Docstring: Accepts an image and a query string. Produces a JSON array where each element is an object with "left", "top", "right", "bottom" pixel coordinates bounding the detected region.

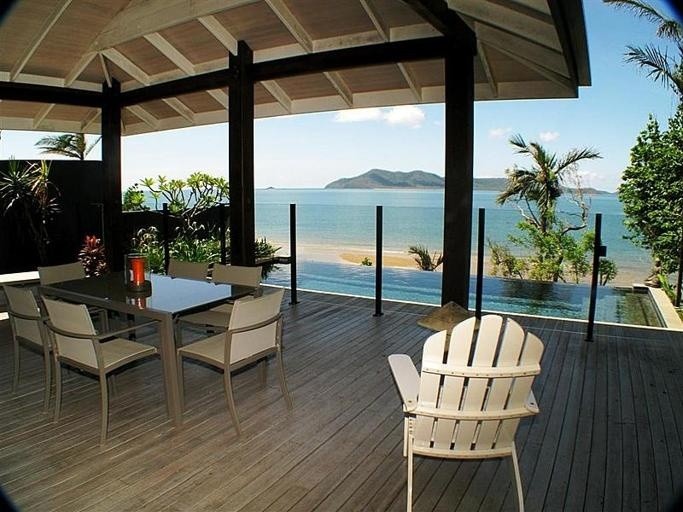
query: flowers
[{"left": 79, "top": 235, "right": 107, "bottom": 280}]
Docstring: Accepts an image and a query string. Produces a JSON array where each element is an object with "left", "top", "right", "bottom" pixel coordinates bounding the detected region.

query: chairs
[
  {"left": 176, "top": 286, "right": 291, "bottom": 438},
  {"left": 37, "top": 260, "right": 105, "bottom": 338},
  {"left": 386, "top": 314, "right": 544, "bottom": 512},
  {"left": 2, "top": 283, "right": 112, "bottom": 414},
  {"left": 39, "top": 294, "right": 168, "bottom": 445},
  {"left": 178, "top": 264, "right": 267, "bottom": 389},
  {"left": 166, "top": 257, "right": 212, "bottom": 348}
]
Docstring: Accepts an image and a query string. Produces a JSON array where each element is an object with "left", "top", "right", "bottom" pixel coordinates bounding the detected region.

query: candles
[{"left": 133, "top": 261, "right": 145, "bottom": 284}]
